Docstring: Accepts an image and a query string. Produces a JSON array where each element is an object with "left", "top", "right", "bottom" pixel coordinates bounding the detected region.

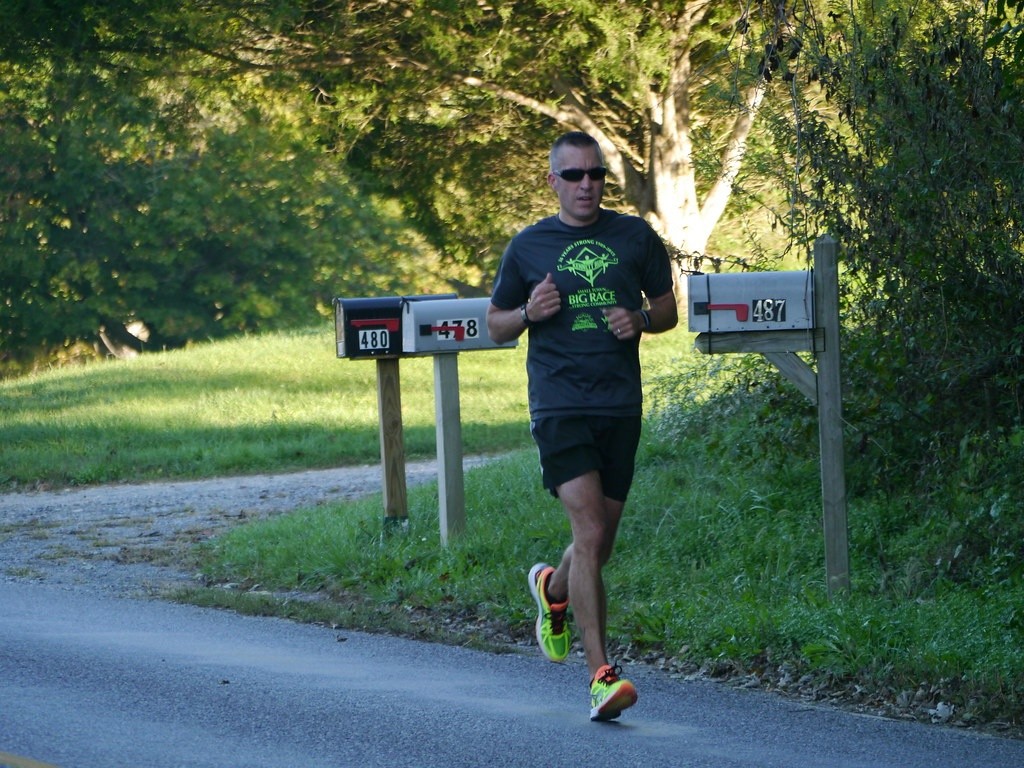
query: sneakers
[
  {"left": 528, "top": 563, "right": 571, "bottom": 662},
  {"left": 590, "top": 660, "right": 637, "bottom": 722}
]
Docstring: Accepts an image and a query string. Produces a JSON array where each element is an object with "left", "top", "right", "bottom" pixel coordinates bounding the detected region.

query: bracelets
[{"left": 638, "top": 309, "right": 651, "bottom": 331}]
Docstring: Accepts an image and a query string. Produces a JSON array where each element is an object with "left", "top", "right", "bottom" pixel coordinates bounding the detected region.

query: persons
[{"left": 485, "top": 131, "right": 679, "bottom": 722}]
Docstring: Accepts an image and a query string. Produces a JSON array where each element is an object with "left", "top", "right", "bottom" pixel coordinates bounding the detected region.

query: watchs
[{"left": 520, "top": 303, "right": 539, "bottom": 326}]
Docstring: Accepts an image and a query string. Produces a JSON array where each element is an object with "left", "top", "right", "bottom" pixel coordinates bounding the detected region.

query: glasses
[{"left": 552, "top": 167, "right": 607, "bottom": 181}]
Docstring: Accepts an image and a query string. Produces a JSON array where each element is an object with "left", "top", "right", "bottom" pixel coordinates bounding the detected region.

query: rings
[{"left": 617, "top": 328, "right": 621, "bottom": 334}]
